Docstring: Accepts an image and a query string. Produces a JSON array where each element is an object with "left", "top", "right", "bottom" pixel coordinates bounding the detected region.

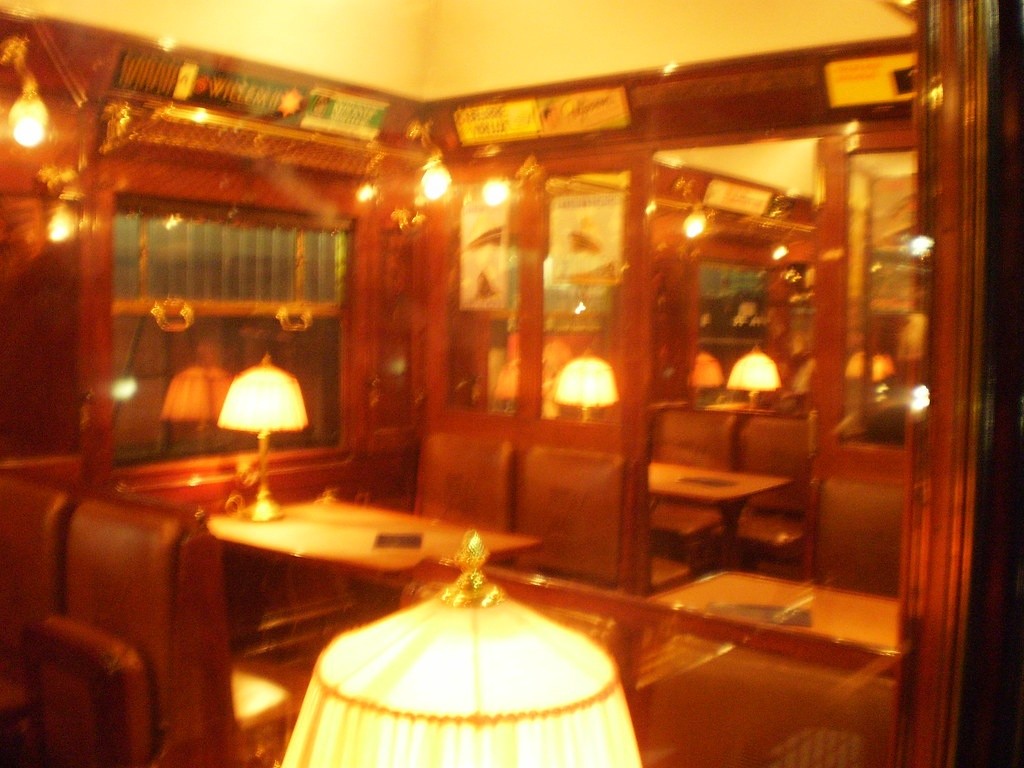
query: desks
[
  {"left": 206, "top": 495, "right": 541, "bottom": 667},
  {"left": 647, "top": 459, "right": 795, "bottom": 571},
  {"left": 615, "top": 572, "right": 902, "bottom": 768}
]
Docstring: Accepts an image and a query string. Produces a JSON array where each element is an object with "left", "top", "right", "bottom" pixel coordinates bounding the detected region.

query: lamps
[
  {"left": 162, "top": 366, "right": 230, "bottom": 454},
  {"left": 729, "top": 347, "right": 779, "bottom": 411},
  {"left": 693, "top": 352, "right": 722, "bottom": 391},
  {"left": 10, "top": 95, "right": 47, "bottom": 149},
  {"left": 553, "top": 356, "right": 617, "bottom": 425},
  {"left": 277, "top": 530, "right": 645, "bottom": 768},
  {"left": 422, "top": 158, "right": 451, "bottom": 201},
  {"left": 218, "top": 355, "right": 308, "bottom": 523}
]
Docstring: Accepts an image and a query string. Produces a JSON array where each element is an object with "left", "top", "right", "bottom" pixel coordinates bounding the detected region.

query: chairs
[
  {"left": 739, "top": 415, "right": 817, "bottom": 580},
  {"left": 7, "top": 617, "right": 150, "bottom": 767},
  {"left": 414, "top": 431, "right": 513, "bottom": 535},
  {"left": 0, "top": 473, "right": 67, "bottom": 715},
  {"left": 648, "top": 411, "right": 735, "bottom": 577},
  {"left": 519, "top": 445, "right": 621, "bottom": 587},
  {"left": 60, "top": 492, "right": 290, "bottom": 767}
]
